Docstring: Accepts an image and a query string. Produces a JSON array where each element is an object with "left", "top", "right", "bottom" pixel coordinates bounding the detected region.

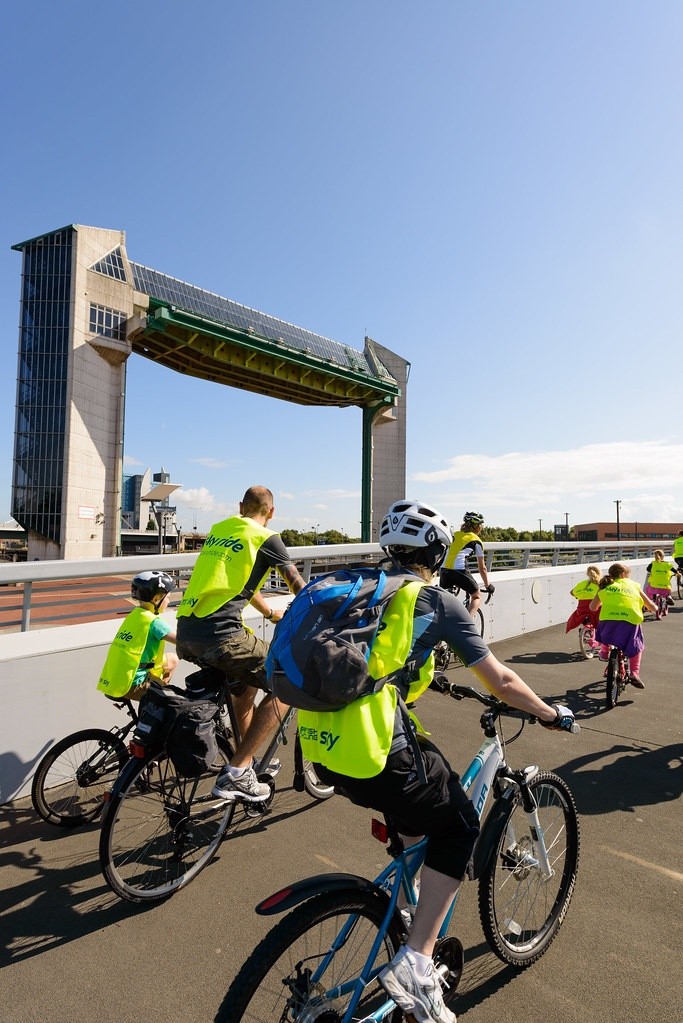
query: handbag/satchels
[{"left": 135, "top": 680, "right": 220, "bottom": 777}]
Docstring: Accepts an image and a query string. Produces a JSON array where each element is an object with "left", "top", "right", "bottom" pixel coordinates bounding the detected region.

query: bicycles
[
  {"left": 678, "top": 565, "right": 683, "bottom": 600},
  {"left": 605, "top": 645, "right": 637, "bottom": 708},
  {"left": 656, "top": 573, "right": 678, "bottom": 620},
  {"left": 580, "top": 620, "right": 600, "bottom": 660},
  {"left": 215, "top": 681, "right": 581, "bottom": 1023},
  {"left": 31, "top": 686, "right": 336, "bottom": 906},
  {"left": 435, "top": 588, "right": 492, "bottom": 672}
]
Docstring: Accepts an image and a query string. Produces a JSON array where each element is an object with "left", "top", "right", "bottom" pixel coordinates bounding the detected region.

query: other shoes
[
  {"left": 630, "top": 676, "right": 645, "bottom": 689},
  {"left": 666, "top": 597, "right": 674, "bottom": 606}
]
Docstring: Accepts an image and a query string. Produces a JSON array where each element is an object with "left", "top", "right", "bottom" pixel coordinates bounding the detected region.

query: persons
[
  {"left": 439, "top": 511, "right": 495, "bottom": 619},
  {"left": 96, "top": 571, "right": 179, "bottom": 704},
  {"left": 570, "top": 567, "right": 604, "bottom": 648},
  {"left": 590, "top": 564, "right": 658, "bottom": 689},
  {"left": 297, "top": 500, "right": 574, "bottom": 1023},
  {"left": 174, "top": 486, "right": 306, "bottom": 802},
  {"left": 646, "top": 550, "right": 682, "bottom": 602},
  {"left": 673, "top": 530, "right": 683, "bottom": 572}
]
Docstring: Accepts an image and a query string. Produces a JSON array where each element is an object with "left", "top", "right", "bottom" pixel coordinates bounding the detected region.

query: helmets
[
  {"left": 464, "top": 511, "right": 484, "bottom": 523},
  {"left": 131, "top": 571, "right": 177, "bottom": 601},
  {"left": 379, "top": 499, "right": 454, "bottom": 547}
]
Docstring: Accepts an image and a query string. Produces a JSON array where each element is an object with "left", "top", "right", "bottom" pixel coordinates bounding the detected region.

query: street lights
[
  {"left": 312, "top": 523, "right": 320, "bottom": 545},
  {"left": 302, "top": 530, "right": 305, "bottom": 546}
]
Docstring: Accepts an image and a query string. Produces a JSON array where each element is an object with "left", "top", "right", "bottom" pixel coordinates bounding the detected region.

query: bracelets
[{"left": 264, "top": 609, "right": 274, "bottom": 619}]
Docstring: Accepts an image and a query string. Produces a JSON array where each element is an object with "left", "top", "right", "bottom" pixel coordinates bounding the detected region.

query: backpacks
[{"left": 266, "top": 569, "right": 434, "bottom": 711}]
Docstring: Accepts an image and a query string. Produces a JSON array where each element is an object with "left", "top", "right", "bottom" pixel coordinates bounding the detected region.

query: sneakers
[
  {"left": 211, "top": 765, "right": 272, "bottom": 802},
  {"left": 378, "top": 945, "right": 457, "bottom": 1023},
  {"left": 399, "top": 880, "right": 419, "bottom": 935},
  {"left": 253, "top": 758, "right": 281, "bottom": 777}
]
